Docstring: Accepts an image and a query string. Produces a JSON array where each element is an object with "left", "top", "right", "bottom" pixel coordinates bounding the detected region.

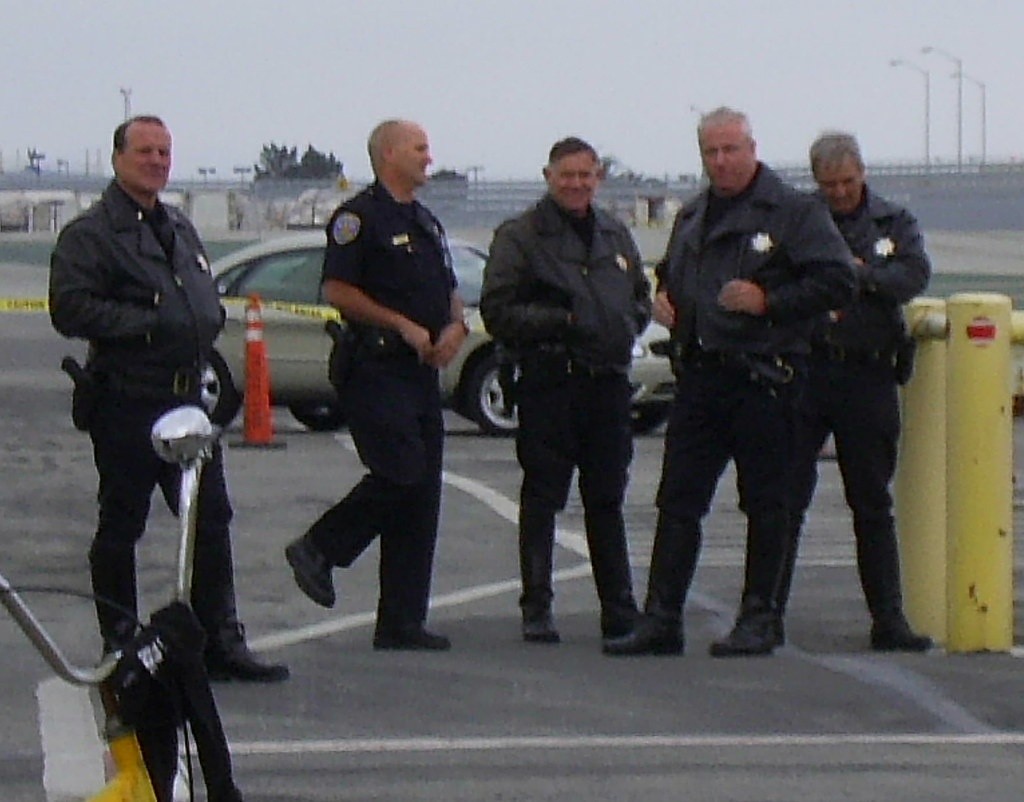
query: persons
[
  {"left": 48, "top": 114, "right": 290, "bottom": 683},
  {"left": 284, "top": 117, "right": 464, "bottom": 652},
  {"left": 770, "top": 131, "right": 935, "bottom": 657},
  {"left": 604, "top": 107, "right": 859, "bottom": 659},
  {"left": 482, "top": 136, "right": 651, "bottom": 656}
]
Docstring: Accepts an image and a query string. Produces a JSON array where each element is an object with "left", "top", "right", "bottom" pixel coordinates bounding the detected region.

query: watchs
[{"left": 452, "top": 318, "right": 471, "bottom": 334}]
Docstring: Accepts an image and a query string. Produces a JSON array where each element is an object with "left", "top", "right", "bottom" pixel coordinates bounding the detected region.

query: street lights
[
  {"left": 199, "top": 168, "right": 216, "bottom": 183},
  {"left": 892, "top": 60, "right": 930, "bottom": 174},
  {"left": 950, "top": 73, "right": 986, "bottom": 173},
  {"left": 234, "top": 168, "right": 251, "bottom": 189},
  {"left": 923, "top": 47, "right": 962, "bottom": 173}
]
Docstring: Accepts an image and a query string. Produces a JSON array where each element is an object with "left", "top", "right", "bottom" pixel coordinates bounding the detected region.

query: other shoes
[
  {"left": 522, "top": 606, "right": 560, "bottom": 643},
  {"left": 207, "top": 641, "right": 289, "bottom": 682},
  {"left": 604, "top": 626, "right": 684, "bottom": 656},
  {"left": 287, "top": 533, "right": 339, "bottom": 608},
  {"left": 871, "top": 631, "right": 933, "bottom": 653},
  {"left": 373, "top": 624, "right": 451, "bottom": 651},
  {"left": 600, "top": 611, "right": 639, "bottom": 636},
  {"left": 709, "top": 629, "right": 776, "bottom": 658}
]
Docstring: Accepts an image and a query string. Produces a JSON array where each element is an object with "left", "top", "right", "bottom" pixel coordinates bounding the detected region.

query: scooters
[{"left": 1, "top": 404, "right": 213, "bottom": 802}]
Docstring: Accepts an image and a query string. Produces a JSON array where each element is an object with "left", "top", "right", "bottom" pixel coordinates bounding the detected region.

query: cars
[{"left": 200, "top": 231, "right": 677, "bottom": 439}]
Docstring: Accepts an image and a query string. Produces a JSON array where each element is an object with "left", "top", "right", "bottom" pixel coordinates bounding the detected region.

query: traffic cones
[{"left": 226, "top": 294, "right": 286, "bottom": 449}]
